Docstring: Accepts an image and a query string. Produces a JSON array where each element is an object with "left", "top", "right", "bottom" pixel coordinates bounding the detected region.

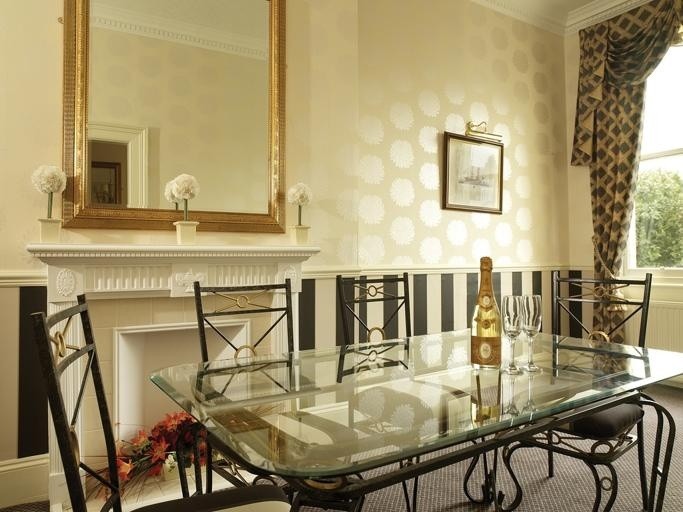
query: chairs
[
  {"left": 28, "top": 293, "right": 296, "bottom": 512},
  {"left": 495, "top": 269, "right": 652, "bottom": 512},
  {"left": 193, "top": 280, "right": 365, "bottom": 512},
  {"left": 339, "top": 273, "right": 494, "bottom": 512}
]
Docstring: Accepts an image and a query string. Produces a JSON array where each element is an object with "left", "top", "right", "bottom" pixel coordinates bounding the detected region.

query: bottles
[{"left": 469, "top": 255, "right": 502, "bottom": 371}]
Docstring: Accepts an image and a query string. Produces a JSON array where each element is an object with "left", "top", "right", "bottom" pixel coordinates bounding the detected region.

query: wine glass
[
  {"left": 521, "top": 294, "right": 543, "bottom": 373},
  {"left": 500, "top": 295, "right": 523, "bottom": 376}
]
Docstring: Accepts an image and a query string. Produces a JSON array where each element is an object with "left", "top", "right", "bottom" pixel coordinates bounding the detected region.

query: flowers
[
  {"left": 164, "top": 179, "right": 183, "bottom": 211},
  {"left": 173, "top": 173, "right": 198, "bottom": 220},
  {"left": 287, "top": 183, "right": 312, "bottom": 226},
  {"left": 30, "top": 164, "right": 67, "bottom": 217}
]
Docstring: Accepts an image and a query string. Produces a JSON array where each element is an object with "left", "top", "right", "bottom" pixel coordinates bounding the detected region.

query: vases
[
  {"left": 172, "top": 220, "right": 199, "bottom": 246},
  {"left": 35, "top": 218, "right": 64, "bottom": 244},
  {"left": 290, "top": 226, "right": 311, "bottom": 244}
]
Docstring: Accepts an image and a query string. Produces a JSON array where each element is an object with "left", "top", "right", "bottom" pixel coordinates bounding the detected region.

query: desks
[{"left": 148, "top": 328, "right": 683, "bottom": 512}]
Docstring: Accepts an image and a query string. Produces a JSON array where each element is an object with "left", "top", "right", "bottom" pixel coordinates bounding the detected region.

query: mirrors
[{"left": 61, "top": 0, "right": 287, "bottom": 234}]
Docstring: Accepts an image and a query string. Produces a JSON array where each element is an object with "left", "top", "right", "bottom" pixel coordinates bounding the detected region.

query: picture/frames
[
  {"left": 440, "top": 131, "right": 504, "bottom": 217},
  {"left": 90, "top": 161, "right": 121, "bottom": 204}
]
